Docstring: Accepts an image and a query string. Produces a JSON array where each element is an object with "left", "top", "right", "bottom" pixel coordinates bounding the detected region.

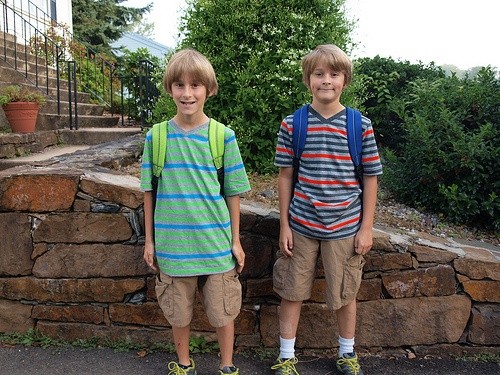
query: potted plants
[{"left": 0, "top": 84, "right": 44, "bottom": 133}]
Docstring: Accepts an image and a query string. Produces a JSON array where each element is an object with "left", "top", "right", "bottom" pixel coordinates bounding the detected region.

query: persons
[
  {"left": 270, "top": 45, "right": 383, "bottom": 375},
  {"left": 140, "top": 49, "right": 251, "bottom": 375}
]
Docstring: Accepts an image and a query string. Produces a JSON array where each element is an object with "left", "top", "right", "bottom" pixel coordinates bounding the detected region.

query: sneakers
[
  {"left": 217, "top": 360, "right": 240, "bottom": 375},
  {"left": 168, "top": 357, "right": 197, "bottom": 374},
  {"left": 336, "top": 352, "right": 364, "bottom": 375},
  {"left": 271, "top": 356, "right": 299, "bottom": 374}
]
